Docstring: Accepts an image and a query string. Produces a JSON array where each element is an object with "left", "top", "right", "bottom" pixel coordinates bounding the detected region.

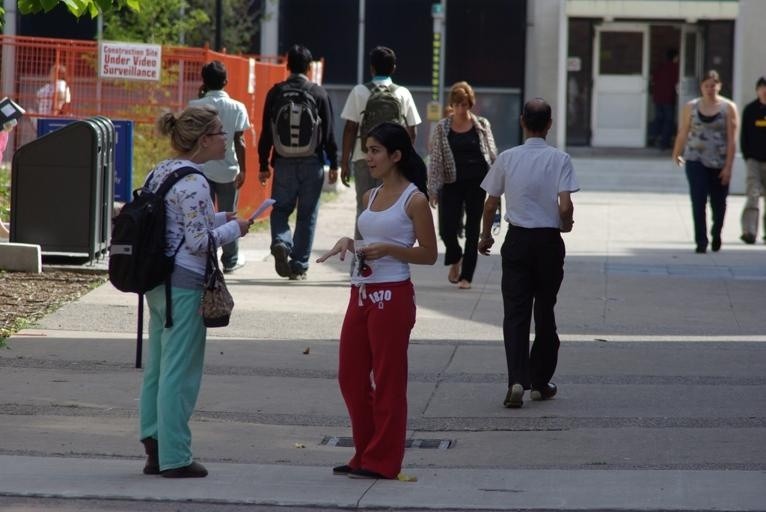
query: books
[{"left": 0, "top": 96, "right": 25, "bottom": 131}]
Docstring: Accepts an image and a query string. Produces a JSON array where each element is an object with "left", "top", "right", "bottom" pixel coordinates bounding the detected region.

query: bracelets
[{"left": 479, "top": 233, "right": 492, "bottom": 242}]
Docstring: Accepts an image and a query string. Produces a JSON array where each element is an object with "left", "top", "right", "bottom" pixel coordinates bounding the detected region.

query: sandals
[{"left": 448, "top": 255, "right": 464, "bottom": 284}]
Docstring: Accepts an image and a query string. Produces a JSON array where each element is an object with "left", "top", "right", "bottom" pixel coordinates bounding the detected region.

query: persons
[
  {"left": 314, "top": 122, "right": 436, "bottom": 481},
  {"left": 429, "top": 81, "right": 496, "bottom": 289},
  {"left": 257, "top": 45, "right": 338, "bottom": 281},
  {"left": 673, "top": 69, "right": 737, "bottom": 254},
  {"left": 27, "top": 63, "right": 71, "bottom": 131},
  {"left": 0, "top": 117, "right": 17, "bottom": 167},
  {"left": 136, "top": 105, "right": 251, "bottom": 479},
  {"left": 738, "top": 75, "right": 766, "bottom": 245},
  {"left": 339, "top": 46, "right": 421, "bottom": 242},
  {"left": 184, "top": 60, "right": 250, "bottom": 274},
  {"left": 478, "top": 98, "right": 580, "bottom": 409}
]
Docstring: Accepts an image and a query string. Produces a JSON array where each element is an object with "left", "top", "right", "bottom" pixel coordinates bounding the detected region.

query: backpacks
[
  {"left": 109, "top": 167, "right": 204, "bottom": 293},
  {"left": 360, "top": 80, "right": 404, "bottom": 151},
  {"left": 268, "top": 80, "right": 323, "bottom": 159}
]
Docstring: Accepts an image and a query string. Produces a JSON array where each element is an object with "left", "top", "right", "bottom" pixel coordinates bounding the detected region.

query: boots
[{"left": 143, "top": 438, "right": 208, "bottom": 477}]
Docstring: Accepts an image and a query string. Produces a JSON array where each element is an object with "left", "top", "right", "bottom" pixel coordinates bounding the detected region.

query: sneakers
[
  {"left": 332, "top": 464, "right": 385, "bottom": 479},
  {"left": 504, "top": 382, "right": 524, "bottom": 408},
  {"left": 223, "top": 256, "right": 247, "bottom": 272},
  {"left": 531, "top": 382, "right": 557, "bottom": 399},
  {"left": 272, "top": 241, "right": 307, "bottom": 280}
]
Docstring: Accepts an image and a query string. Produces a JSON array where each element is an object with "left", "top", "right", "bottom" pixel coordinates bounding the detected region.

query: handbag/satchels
[{"left": 200, "top": 229, "right": 233, "bottom": 327}]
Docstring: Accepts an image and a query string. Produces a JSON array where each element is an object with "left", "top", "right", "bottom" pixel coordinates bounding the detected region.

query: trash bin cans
[{"left": 8, "top": 116, "right": 117, "bottom": 256}]
